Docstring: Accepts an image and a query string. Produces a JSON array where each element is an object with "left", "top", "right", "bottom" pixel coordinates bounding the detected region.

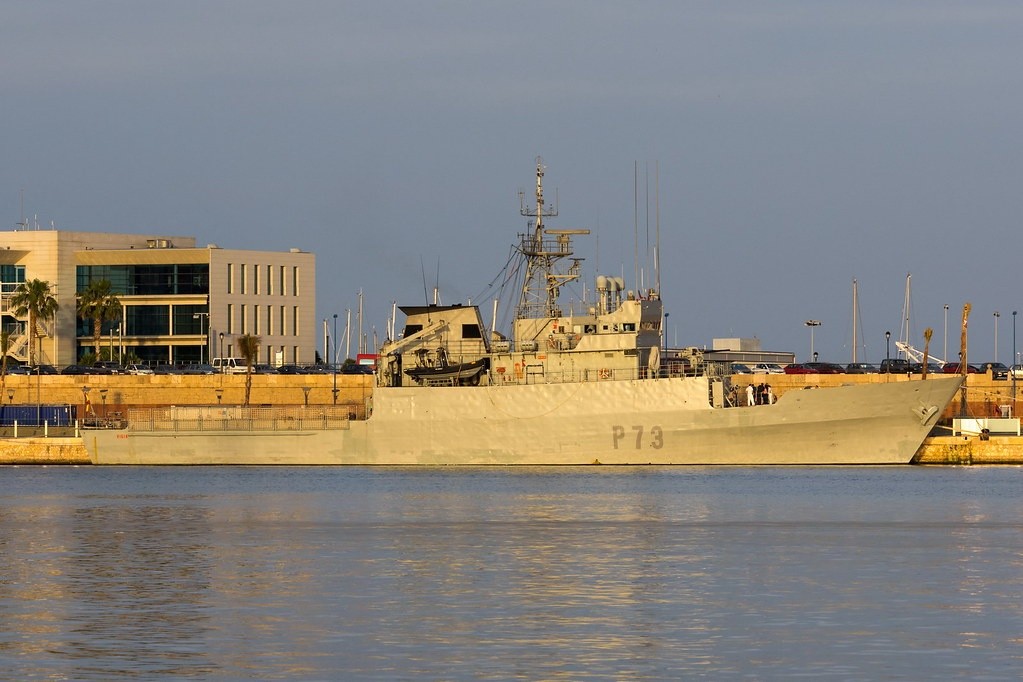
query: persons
[{"left": 745, "top": 383, "right": 772, "bottom": 406}]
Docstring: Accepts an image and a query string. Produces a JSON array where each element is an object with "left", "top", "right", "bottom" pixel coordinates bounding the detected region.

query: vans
[{"left": 212, "top": 358, "right": 256, "bottom": 374}]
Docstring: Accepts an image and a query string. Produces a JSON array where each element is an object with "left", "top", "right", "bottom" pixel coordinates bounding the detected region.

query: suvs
[
  {"left": 879, "top": 358, "right": 914, "bottom": 374},
  {"left": 92, "top": 361, "right": 130, "bottom": 375}
]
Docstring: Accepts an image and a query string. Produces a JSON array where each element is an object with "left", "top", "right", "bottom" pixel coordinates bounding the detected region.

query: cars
[
  {"left": 731, "top": 363, "right": 754, "bottom": 375},
  {"left": 124, "top": 364, "right": 156, "bottom": 375},
  {"left": 61, "top": 364, "right": 100, "bottom": 375},
  {"left": 1010, "top": 364, "right": 1023, "bottom": 379},
  {"left": 979, "top": 362, "right": 1011, "bottom": 380},
  {"left": 154, "top": 365, "right": 184, "bottom": 375},
  {"left": 277, "top": 366, "right": 308, "bottom": 375},
  {"left": 252, "top": 365, "right": 280, "bottom": 374},
  {"left": 4, "top": 366, "right": 38, "bottom": 375},
  {"left": 846, "top": 363, "right": 877, "bottom": 373},
  {"left": 942, "top": 363, "right": 979, "bottom": 375},
  {"left": 303, "top": 364, "right": 344, "bottom": 374},
  {"left": 784, "top": 362, "right": 846, "bottom": 375},
  {"left": 340, "top": 364, "right": 376, "bottom": 374},
  {"left": 751, "top": 363, "right": 786, "bottom": 374},
  {"left": 912, "top": 362, "right": 942, "bottom": 373},
  {"left": 182, "top": 364, "right": 220, "bottom": 375},
  {"left": 29, "top": 365, "right": 60, "bottom": 374}
]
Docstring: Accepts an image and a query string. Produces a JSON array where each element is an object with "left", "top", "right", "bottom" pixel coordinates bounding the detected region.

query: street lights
[
  {"left": 993, "top": 311, "right": 1000, "bottom": 362},
  {"left": 219, "top": 331, "right": 224, "bottom": 374},
  {"left": 194, "top": 313, "right": 211, "bottom": 365},
  {"left": 806, "top": 319, "right": 822, "bottom": 363},
  {"left": 943, "top": 303, "right": 949, "bottom": 362},
  {"left": 664, "top": 313, "right": 669, "bottom": 366},
  {"left": 332, "top": 314, "right": 338, "bottom": 406},
  {"left": 1012, "top": 311, "right": 1017, "bottom": 416}
]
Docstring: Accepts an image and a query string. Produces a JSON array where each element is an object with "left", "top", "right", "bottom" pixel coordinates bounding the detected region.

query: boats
[
  {"left": 402, "top": 355, "right": 491, "bottom": 381},
  {"left": 76, "top": 154, "right": 970, "bottom": 466}
]
